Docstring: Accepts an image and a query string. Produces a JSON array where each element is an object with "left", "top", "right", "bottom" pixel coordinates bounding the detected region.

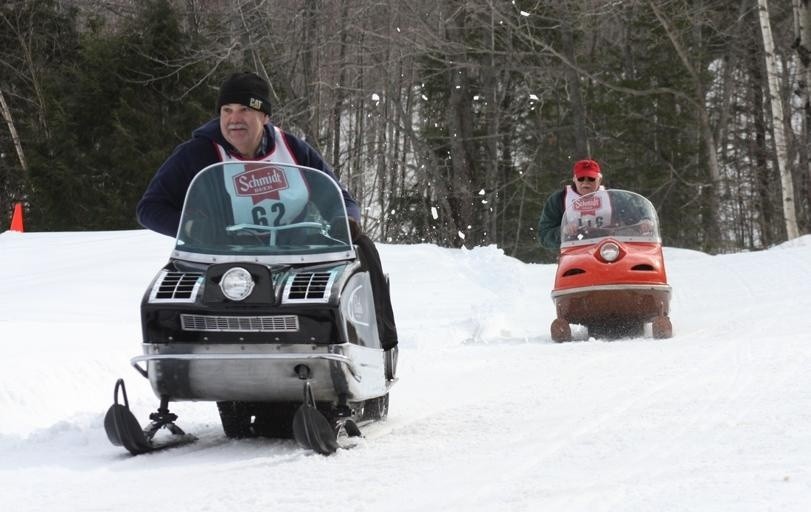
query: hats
[
  {"left": 219, "top": 72, "right": 273, "bottom": 115},
  {"left": 574, "top": 159, "right": 601, "bottom": 178}
]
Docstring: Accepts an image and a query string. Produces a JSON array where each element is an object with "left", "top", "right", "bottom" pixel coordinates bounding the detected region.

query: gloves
[
  {"left": 191, "top": 221, "right": 224, "bottom": 246},
  {"left": 329, "top": 217, "right": 361, "bottom": 246}
]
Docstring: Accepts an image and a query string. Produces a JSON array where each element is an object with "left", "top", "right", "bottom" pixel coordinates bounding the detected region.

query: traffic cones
[{"left": 10, "top": 202, "right": 24, "bottom": 232}]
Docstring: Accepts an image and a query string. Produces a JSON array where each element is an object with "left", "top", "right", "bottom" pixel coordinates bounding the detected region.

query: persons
[
  {"left": 135, "top": 71, "right": 364, "bottom": 246},
  {"left": 535, "top": 160, "right": 655, "bottom": 252}
]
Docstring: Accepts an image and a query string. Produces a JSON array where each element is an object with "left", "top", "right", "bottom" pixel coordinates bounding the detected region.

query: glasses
[{"left": 578, "top": 177, "right": 595, "bottom": 183}]
